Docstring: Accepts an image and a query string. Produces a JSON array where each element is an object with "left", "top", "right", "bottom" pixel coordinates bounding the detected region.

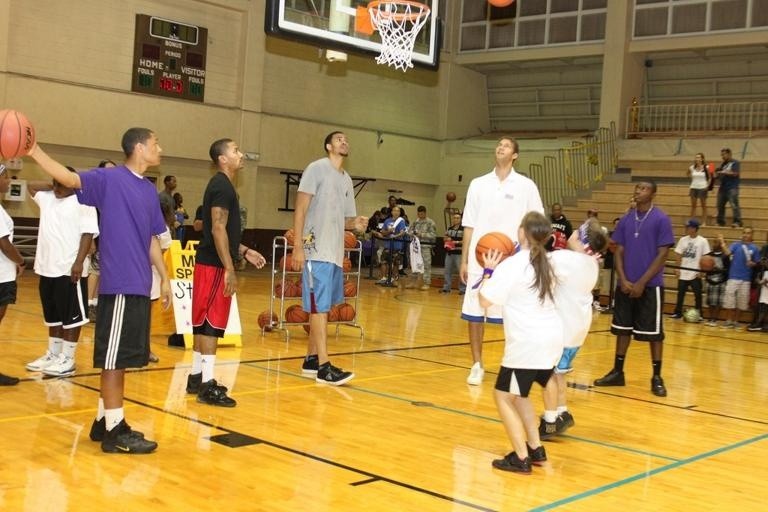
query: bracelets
[
  {"left": 242, "top": 248, "right": 250, "bottom": 260},
  {"left": 20, "top": 258, "right": 26, "bottom": 267},
  {"left": 482, "top": 267, "right": 493, "bottom": 280}
]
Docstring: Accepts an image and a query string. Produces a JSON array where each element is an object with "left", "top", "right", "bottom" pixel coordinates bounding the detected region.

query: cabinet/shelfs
[{"left": 261, "top": 234, "right": 367, "bottom": 341}]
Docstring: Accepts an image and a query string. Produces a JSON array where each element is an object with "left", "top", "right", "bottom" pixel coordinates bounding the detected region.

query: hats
[{"left": 685, "top": 219, "right": 699, "bottom": 226}]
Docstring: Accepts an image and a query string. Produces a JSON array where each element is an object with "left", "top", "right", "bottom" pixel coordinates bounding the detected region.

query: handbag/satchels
[{"left": 704, "top": 163, "right": 714, "bottom": 191}]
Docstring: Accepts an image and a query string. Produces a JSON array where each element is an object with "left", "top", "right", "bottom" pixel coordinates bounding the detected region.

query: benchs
[{"left": 555, "top": 159, "right": 768, "bottom": 324}]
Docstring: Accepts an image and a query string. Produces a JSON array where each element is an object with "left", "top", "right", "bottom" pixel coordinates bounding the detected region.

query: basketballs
[
  {"left": 0, "top": 109, "right": 36, "bottom": 160},
  {"left": 488, "top": 0, "right": 514, "bottom": 8},
  {"left": 258, "top": 228, "right": 357, "bottom": 335},
  {"left": 700, "top": 256, "right": 715, "bottom": 270},
  {"left": 683, "top": 308, "right": 701, "bottom": 323},
  {"left": 475, "top": 233, "right": 514, "bottom": 268},
  {"left": 446, "top": 192, "right": 456, "bottom": 202}
]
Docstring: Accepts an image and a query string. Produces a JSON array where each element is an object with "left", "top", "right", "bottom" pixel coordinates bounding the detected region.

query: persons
[
  {"left": 25, "top": 158, "right": 203, "bottom": 377},
  {"left": 593, "top": 179, "right": 676, "bottom": 397},
  {"left": 687, "top": 152, "right": 714, "bottom": 227},
  {"left": 674, "top": 219, "right": 768, "bottom": 334},
  {"left": 290, "top": 128, "right": 369, "bottom": 384},
  {"left": 537, "top": 216, "right": 609, "bottom": 439},
  {"left": 713, "top": 148, "right": 744, "bottom": 227},
  {"left": 549, "top": 201, "right": 620, "bottom": 311},
  {"left": 24, "top": 125, "right": 176, "bottom": 457},
  {"left": 477, "top": 211, "right": 566, "bottom": 475},
  {"left": 23, "top": 372, "right": 86, "bottom": 512},
  {"left": 663, "top": 327, "right": 768, "bottom": 444},
  {"left": 368, "top": 195, "right": 466, "bottom": 295},
  {"left": 458, "top": 135, "right": 547, "bottom": 385},
  {"left": 0, "top": 163, "right": 25, "bottom": 383},
  {"left": 186, "top": 139, "right": 266, "bottom": 406}
]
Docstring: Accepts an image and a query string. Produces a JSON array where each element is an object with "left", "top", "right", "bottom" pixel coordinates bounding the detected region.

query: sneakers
[
  {"left": 539, "top": 411, "right": 574, "bottom": 440},
  {"left": 186, "top": 372, "right": 228, "bottom": 394},
  {"left": 0, "top": 352, "right": 77, "bottom": 386},
  {"left": 671, "top": 314, "right": 767, "bottom": 332},
  {"left": 198, "top": 379, "right": 236, "bottom": 407},
  {"left": 301, "top": 358, "right": 319, "bottom": 374},
  {"left": 594, "top": 369, "right": 625, "bottom": 386},
  {"left": 88, "top": 304, "right": 98, "bottom": 322},
  {"left": 491, "top": 442, "right": 547, "bottom": 475},
  {"left": 651, "top": 375, "right": 666, "bottom": 396},
  {"left": 316, "top": 363, "right": 355, "bottom": 386},
  {"left": 375, "top": 270, "right": 464, "bottom": 295},
  {"left": 731, "top": 224, "right": 739, "bottom": 227},
  {"left": 90, "top": 416, "right": 157, "bottom": 453},
  {"left": 466, "top": 362, "right": 483, "bottom": 384},
  {"left": 592, "top": 302, "right": 615, "bottom": 314}
]
